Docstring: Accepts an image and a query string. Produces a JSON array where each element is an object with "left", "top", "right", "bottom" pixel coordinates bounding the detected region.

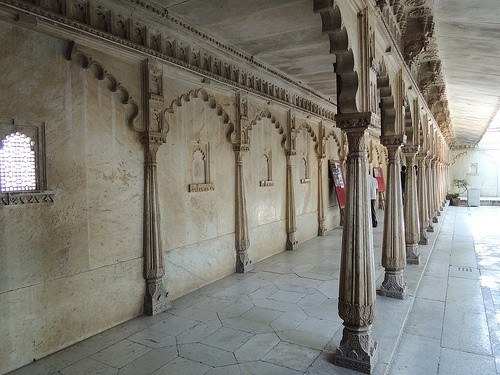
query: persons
[
  {"left": 399, "top": 165, "right": 408, "bottom": 208},
  {"left": 367, "top": 168, "right": 379, "bottom": 227}
]
